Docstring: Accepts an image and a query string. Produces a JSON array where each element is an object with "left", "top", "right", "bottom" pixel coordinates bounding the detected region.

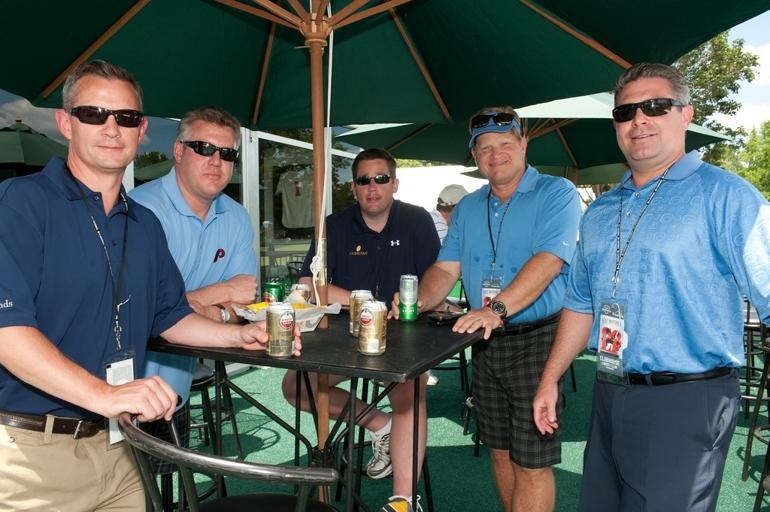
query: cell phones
[{"left": 427, "top": 312, "right": 465, "bottom": 325}]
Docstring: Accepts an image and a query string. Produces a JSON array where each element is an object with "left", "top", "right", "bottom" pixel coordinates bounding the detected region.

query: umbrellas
[
  {"left": 456, "top": 143, "right": 693, "bottom": 197},
  {"left": 0, "top": 1, "right": 770, "bottom": 506},
  {"left": 330, "top": 84, "right": 736, "bottom": 169}
]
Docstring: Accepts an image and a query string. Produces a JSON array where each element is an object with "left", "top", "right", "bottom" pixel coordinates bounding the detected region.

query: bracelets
[{"left": 211, "top": 301, "right": 233, "bottom": 325}]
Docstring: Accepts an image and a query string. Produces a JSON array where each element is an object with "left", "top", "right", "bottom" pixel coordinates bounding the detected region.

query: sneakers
[
  {"left": 366, "top": 430, "right": 392, "bottom": 481},
  {"left": 379, "top": 495, "right": 424, "bottom": 512}
]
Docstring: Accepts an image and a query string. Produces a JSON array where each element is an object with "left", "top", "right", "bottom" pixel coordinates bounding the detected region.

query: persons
[
  {"left": 2, "top": 60, "right": 305, "bottom": 512},
  {"left": 122, "top": 106, "right": 265, "bottom": 485},
  {"left": 414, "top": 184, "right": 474, "bottom": 388},
  {"left": 530, "top": 61, "right": 770, "bottom": 511},
  {"left": 385, "top": 104, "right": 584, "bottom": 512},
  {"left": 280, "top": 147, "right": 444, "bottom": 512}
]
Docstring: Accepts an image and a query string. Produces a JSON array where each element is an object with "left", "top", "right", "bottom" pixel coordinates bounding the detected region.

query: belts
[
  {"left": 597, "top": 368, "right": 731, "bottom": 386},
  {"left": 0, "top": 409, "right": 106, "bottom": 439},
  {"left": 494, "top": 312, "right": 561, "bottom": 336}
]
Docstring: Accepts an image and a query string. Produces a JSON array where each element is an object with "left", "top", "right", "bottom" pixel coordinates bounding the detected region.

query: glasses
[
  {"left": 181, "top": 140, "right": 238, "bottom": 162},
  {"left": 354, "top": 174, "right": 392, "bottom": 185},
  {"left": 612, "top": 97, "right": 693, "bottom": 122},
  {"left": 71, "top": 105, "right": 144, "bottom": 127},
  {"left": 471, "top": 112, "right": 519, "bottom": 128}
]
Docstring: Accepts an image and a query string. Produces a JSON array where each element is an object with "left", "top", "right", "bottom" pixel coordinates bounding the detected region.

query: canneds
[
  {"left": 266, "top": 301, "right": 295, "bottom": 360},
  {"left": 261, "top": 280, "right": 283, "bottom": 302},
  {"left": 349, "top": 290, "right": 374, "bottom": 337},
  {"left": 290, "top": 283, "right": 311, "bottom": 303},
  {"left": 356, "top": 298, "right": 388, "bottom": 356},
  {"left": 399, "top": 274, "right": 419, "bottom": 321}
]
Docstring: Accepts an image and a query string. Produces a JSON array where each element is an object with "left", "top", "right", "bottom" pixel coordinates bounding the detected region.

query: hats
[
  {"left": 438, "top": 184, "right": 469, "bottom": 206},
  {"left": 468, "top": 111, "right": 521, "bottom": 147}
]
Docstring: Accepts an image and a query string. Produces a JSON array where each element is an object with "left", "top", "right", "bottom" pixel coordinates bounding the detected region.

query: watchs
[{"left": 488, "top": 297, "right": 508, "bottom": 324}]
